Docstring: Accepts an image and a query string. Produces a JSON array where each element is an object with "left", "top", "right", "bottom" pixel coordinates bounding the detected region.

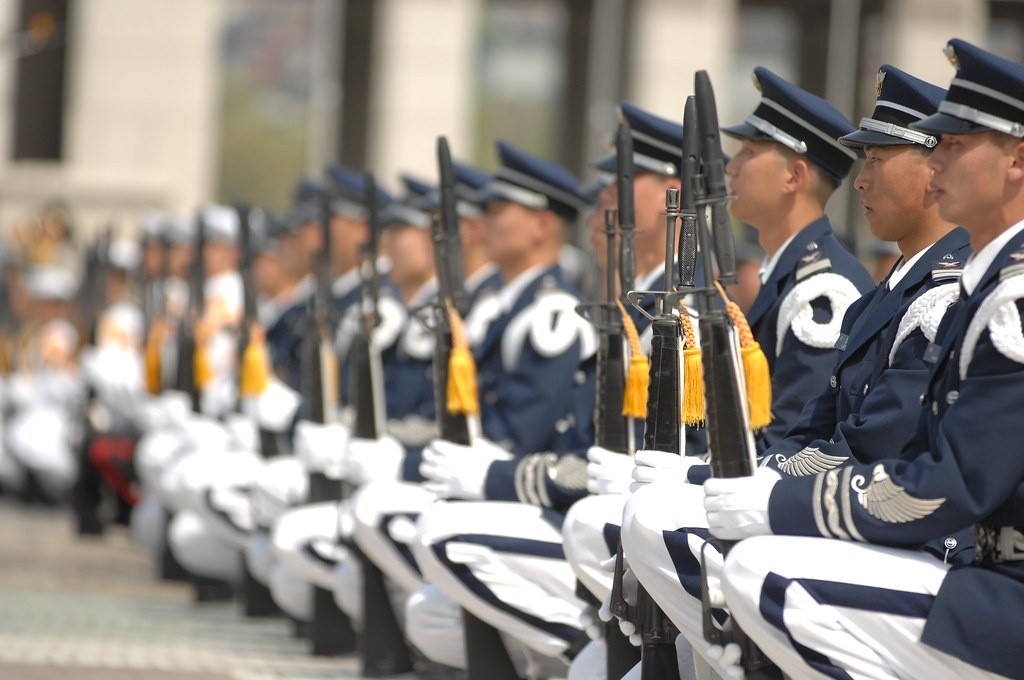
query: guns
[{"left": 74, "top": 66, "right": 786, "bottom": 680}]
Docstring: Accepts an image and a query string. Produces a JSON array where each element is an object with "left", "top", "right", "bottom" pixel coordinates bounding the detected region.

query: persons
[
  {"left": 411, "top": 100, "right": 733, "bottom": 666},
  {"left": 403, "top": 132, "right": 617, "bottom": 678},
  {"left": 702, "top": 37, "right": 1024, "bottom": 680},
  {"left": 598, "top": 65, "right": 973, "bottom": 680},
  {"left": 342, "top": 139, "right": 599, "bottom": 598},
  {"left": 0, "top": 161, "right": 503, "bottom": 622},
  {"left": 563, "top": 66, "right": 876, "bottom": 680}
]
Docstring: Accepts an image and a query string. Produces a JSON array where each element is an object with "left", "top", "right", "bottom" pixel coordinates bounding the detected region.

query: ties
[
  {"left": 954, "top": 277, "right": 969, "bottom": 310},
  {"left": 877, "top": 282, "right": 890, "bottom": 304}
]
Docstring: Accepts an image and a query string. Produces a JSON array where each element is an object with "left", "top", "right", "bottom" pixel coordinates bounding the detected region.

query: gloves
[
  {"left": 418, "top": 436, "right": 515, "bottom": 501},
  {"left": 585, "top": 446, "right": 637, "bottom": 498},
  {"left": 340, "top": 433, "right": 405, "bottom": 488},
  {"left": 629, "top": 449, "right": 705, "bottom": 497},
  {"left": 295, "top": 422, "right": 345, "bottom": 476},
  {"left": 703, "top": 464, "right": 781, "bottom": 542}
]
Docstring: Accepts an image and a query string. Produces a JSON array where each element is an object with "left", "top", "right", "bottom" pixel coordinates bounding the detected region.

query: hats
[
  {"left": 6, "top": 200, "right": 286, "bottom": 303},
  {"left": 837, "top": 64, "right": 949, "bottom": 148},
  {"left": 377, "top": 177, "right": 439, "bottom": 229},
  {"left": 283, "top": 180, "right": 335, "bottom": 229},
  {"left": 719, "top": 66, "right": 867, "bottom": 185},
  {"left": 328, "top": 167, "right": 394, "bottom": 224},
  {"left": 463, "top": 138, "right": 598, "bottom": 224},
  {"left": 908, "top": 38, "right": 1024, "bottom": 139},
  {"left": 409, "top": 165, "right": 492, "bottom": 219},
  {"left": 588, "top": 100, "right": 729, "bottom": 173}
]
